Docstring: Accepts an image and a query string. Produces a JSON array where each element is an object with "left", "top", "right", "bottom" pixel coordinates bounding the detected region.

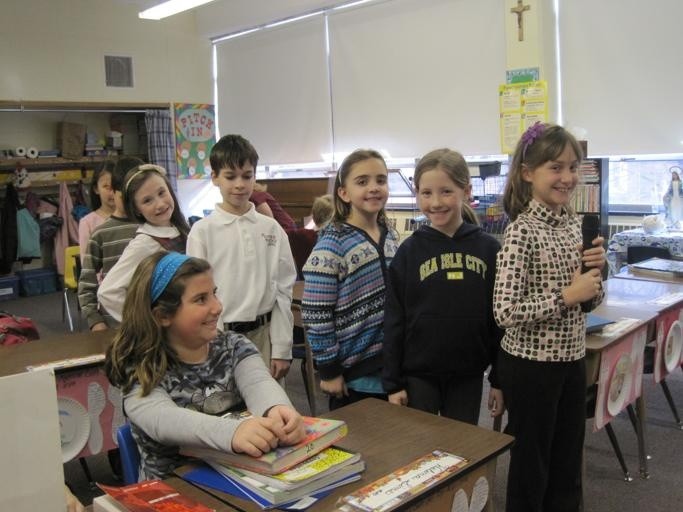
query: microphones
[{"left": 580, "top": 212, "right": 600, "bottom": 313}]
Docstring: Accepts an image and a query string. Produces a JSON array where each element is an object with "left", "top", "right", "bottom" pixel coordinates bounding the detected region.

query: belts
[{"left": 226, "top": 311, "right": 272, "bottom": 334}]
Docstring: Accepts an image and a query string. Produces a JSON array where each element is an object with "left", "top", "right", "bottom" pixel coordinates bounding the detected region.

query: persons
[
  {"left": 245, "top": 178, "right": 299, "bottom": 264},
  {"left": 106, "top": 250, "right": 306, "bottom": 484},
  {"left": 312, "top": 194, "right": 336, "bottom": 228},
  {"left": 301, "top": 150, "right": 401, "bottom": 411},
  {"left": 186, "top": 134, "right": 299, "bottom": 393},
  {"left": 489, "top": 123, "right": 607, "bottom": 512},
  {"left": 95, "top": 163, "right": 190, "bottom": 324},
  {"left": 75, "top": 154, "right": 149, "bottom": 332},
  {"left": 382, "top": 148, "right": 506, "bottom": 426},
  {"left": 665, "top": 172, "right": 683, "bottom": 225},
  {"left": 77, "top": 155, "right": 124, "bottom": 264}
]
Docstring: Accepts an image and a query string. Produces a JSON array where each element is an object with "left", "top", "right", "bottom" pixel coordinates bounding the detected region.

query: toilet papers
[
  {"left": 14, "top": 146, "right": 26, "bottom": 157},
  {"left": 27, "top": 146, "right": 38, "bottom": 159}
]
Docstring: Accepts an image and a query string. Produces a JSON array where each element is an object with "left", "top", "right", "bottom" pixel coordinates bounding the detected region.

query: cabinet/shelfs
[{"left": 568, "top": 157, "right": 611, "bottom": 280}]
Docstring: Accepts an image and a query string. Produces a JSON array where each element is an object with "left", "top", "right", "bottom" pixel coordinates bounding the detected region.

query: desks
[
  {"left": 269, "top": 228, "right": 683, "bottom": 487},
  {"left": 2, "top": 315, "right": 516, "bottom": 512}
]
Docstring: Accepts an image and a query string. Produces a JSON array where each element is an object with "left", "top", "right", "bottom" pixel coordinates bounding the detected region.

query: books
[{"left": 82, "top": 413, "right": 369, "bottom": 512}]
